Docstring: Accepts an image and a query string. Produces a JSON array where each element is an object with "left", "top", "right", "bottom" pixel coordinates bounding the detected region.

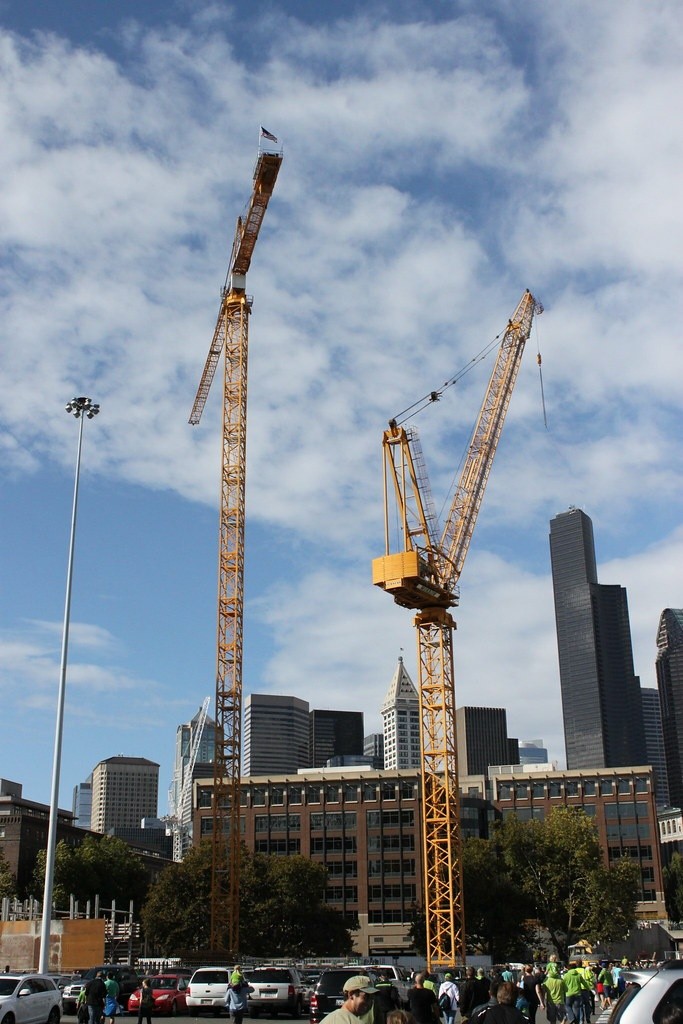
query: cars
[
  {"left": 607, "top": 968, "right": 683, "bottom": 1024},
  {"left": 295, "top": 968, "right": 325, "bottom": 1015},
  {"left": 128, "top": 974, "right": 191, "bottom": 1016},
  {"left": 0, "top": 972, "right": 63, "bottom": 1024},
  {"left": 42, "top": 973, "right": 82, "bottom": 993}
]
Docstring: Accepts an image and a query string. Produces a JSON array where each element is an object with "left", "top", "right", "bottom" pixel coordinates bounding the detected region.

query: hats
[
  {"left": 342, "top": 975, "right": 381, "bottom": 995},
  {"left": 602, "top": 963, "right": 607, "bottom": 968},
  {"left": 445, "top": 973, "right": 453, "bottom": 979}
]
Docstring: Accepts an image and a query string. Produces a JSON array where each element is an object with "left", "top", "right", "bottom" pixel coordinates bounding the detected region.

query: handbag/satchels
[{"left": 437, "top": 993, "right": 453, "bottom": 1011}]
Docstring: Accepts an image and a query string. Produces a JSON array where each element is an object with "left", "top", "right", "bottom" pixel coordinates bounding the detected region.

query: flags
[{"left": 261, "top": 126, "right": 277, "bottom": 144}]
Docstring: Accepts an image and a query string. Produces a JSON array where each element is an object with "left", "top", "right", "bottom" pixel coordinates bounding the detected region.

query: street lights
[{"left": 37, "top": 393, "right": 102, "bottom": 974}]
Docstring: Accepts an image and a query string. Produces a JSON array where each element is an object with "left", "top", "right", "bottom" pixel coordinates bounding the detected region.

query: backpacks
[{"left": 143, "top": 989, "right": 156, "bottom": 1010}]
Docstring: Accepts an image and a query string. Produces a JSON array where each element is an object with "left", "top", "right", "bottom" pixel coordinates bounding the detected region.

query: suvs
[
  {"left": 342, "top": 965, "right": 410, "bottom": 983},
  {"left": 185, "top": 965, "right": 252, "bottom": 1016},
  {"left": 62, "top": 964, "right": 138, "bottom": 1016},
  {"left": 245, "top": 964, "right": 306, "bottom": 1020},
  {"left": 309, "top": 966, "right": 374, "bottom": 1024}
]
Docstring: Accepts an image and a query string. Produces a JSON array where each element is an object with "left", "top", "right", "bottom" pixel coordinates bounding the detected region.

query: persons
[
  {"left": 138, "top": 979, "right": 153, "bottom": 1024},
  {"left": 77, "top": 970, "right": 120, "bottom": 1024},
  {"left": 317, "top": 954, "right": 657, "bottom": 1024},
  {"left": 640, "top": 919, "right": 680, "bottom": 930},
  {"left": 224, "top": 965, "right": 254, "bottom": 1024}
]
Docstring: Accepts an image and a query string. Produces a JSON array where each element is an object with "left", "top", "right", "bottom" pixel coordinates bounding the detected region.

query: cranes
[
  {"left": 371, "top": 288, "right": 546, "bottom": 978},
  {"left": 187, "top": 125, "right": 285, "bottom": 962}
]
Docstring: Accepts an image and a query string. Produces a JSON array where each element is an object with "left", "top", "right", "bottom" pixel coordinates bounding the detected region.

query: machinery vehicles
[{"left": 567, "top": 944, "right": 607, "bottom": 970}]
